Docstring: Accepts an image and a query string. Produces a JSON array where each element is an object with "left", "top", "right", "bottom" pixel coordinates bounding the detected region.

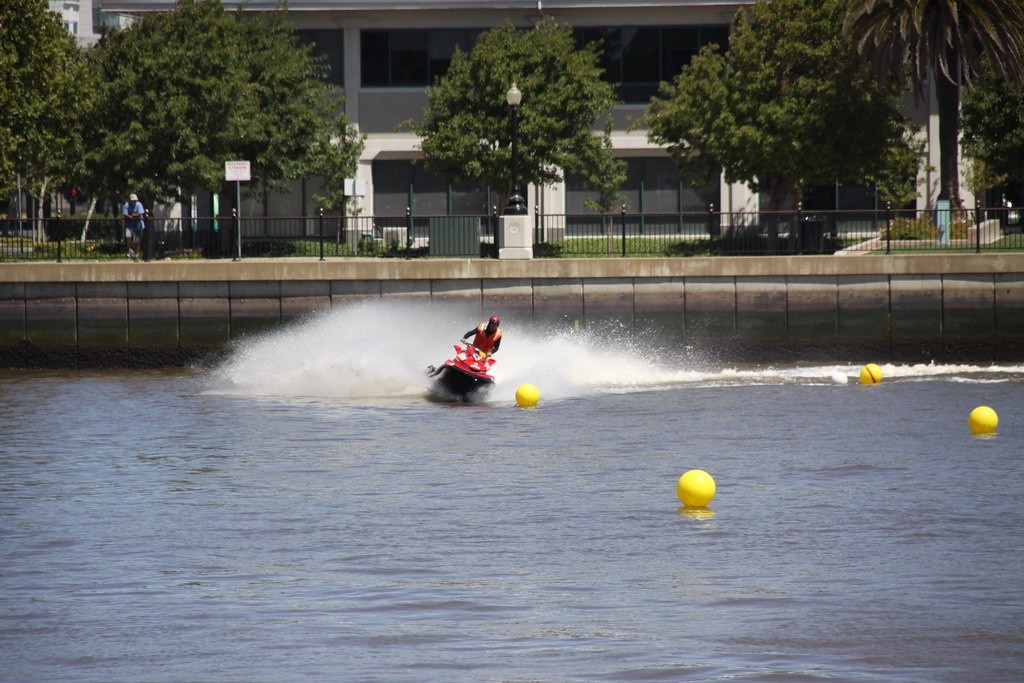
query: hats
[{"left": 129, "top": 193, "right": 138, "bottom": 201}]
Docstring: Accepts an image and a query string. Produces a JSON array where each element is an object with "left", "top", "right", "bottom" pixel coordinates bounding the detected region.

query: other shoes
[{"left": 127, "top": 249, "right": 138, "bottom": 258}]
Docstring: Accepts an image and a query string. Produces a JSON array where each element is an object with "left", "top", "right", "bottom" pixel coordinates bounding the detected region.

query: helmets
[{"left": 490, "top": 315, "right": 499, "bottom": 324}]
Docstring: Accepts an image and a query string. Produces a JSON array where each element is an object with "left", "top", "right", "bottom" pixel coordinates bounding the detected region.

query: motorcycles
[{"left": 424, "top": 342, "right": 497, "bottom": 401}]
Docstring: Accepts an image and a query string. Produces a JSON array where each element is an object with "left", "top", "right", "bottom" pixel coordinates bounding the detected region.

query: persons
[
  {"left": 460, "top": 315, "right": 503, "bottom": 356},
  {"left": 122, "top": 193, "right": 145, "bottom": 262}
]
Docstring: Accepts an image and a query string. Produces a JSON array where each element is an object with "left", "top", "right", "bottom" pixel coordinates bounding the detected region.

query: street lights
[{"left": 503, "top": 78, "right": 529, "bottom": 214}]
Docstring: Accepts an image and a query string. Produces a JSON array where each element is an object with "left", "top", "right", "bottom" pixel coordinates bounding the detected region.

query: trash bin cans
[
  {"left": 362, "top": 233, "right": 372, "bottom": 255},
  {"left": 802, "top": 215, "right": 825, "bottom": 253}
]
